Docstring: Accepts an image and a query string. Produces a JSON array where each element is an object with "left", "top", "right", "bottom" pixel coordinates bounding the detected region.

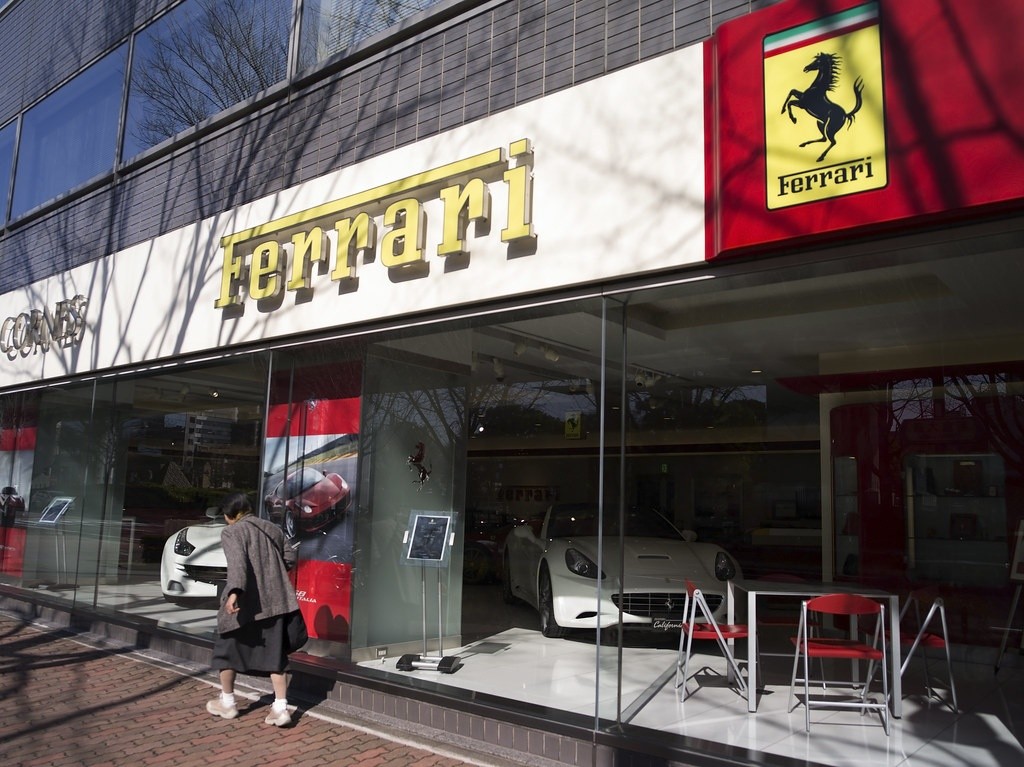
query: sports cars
[{"left": 500, "top": 494, "right": 742, "bottom": 640}]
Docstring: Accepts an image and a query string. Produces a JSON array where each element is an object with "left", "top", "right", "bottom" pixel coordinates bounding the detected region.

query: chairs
[
  {"left": 860, "top": 586, "right": 959, "bottom": 718},
  {"left": 754, "top": 572, "right": 826, "bottom": 690},
  {"left": 674, "top": 578, "right": 763, "bottom": 703},
  {"left": 788, "top": 593, "right": 890, "bottom": 736},
  {"left": 832, "top": 573, "right": 923, "bottom": 698}
]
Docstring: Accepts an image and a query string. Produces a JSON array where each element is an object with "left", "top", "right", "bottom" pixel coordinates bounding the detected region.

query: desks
[{"left": 727, "top": 578, "right": 902, "bottom": 720}]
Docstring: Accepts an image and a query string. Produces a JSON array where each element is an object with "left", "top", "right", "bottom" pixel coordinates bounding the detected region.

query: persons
[{"left": 207, "top": 492, "right": 308, "bottom": 726}]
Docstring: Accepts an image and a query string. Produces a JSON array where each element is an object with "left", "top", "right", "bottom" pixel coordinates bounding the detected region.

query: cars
[
  {"left": 464, "top": 537, "right": 505, "bottom": 585},
  {"left": 263, "top": 466, "right": 354, "bottom": 536},
  {"left": 160, "top": 508, "right": 234, "bottom": 601}
]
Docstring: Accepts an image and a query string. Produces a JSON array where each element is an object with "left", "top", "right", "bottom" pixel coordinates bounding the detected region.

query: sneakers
[
  {"left": 206, "top": 698, "right": 238, "bottom": 721},
  {"left": 265, "top": 707, "right": 291, "bottom": 726}
]
